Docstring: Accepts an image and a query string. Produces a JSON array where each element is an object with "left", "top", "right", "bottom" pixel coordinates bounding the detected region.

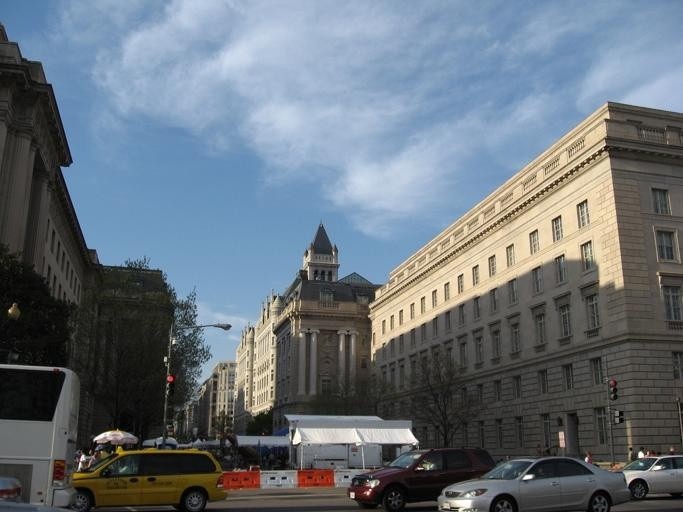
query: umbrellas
[{"left": 92, "top": 427, "right": 139, "bottom": 446}]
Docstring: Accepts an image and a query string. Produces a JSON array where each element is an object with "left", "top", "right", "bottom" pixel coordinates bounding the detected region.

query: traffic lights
[
  {"left": 615, "top": 410, "right": 624, "bottom": 424},
  {"left": 166, "top": 375, "right": 174, "bottom": 396},
  {"left": 609, "top": 380, "right": 618, "bottom": 400}
]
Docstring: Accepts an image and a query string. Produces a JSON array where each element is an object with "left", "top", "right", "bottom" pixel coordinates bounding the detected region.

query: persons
[
  {"left": 72, "top": 443, "right": 130, "bottom": 470},
  {"left": 626, "top": 444, "right": 676, "bottom": 464},
  {"left": 584, "top": 450, "right": 593, "bottom": 465}
]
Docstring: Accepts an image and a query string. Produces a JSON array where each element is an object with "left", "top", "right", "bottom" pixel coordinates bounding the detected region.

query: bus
[{"left": 0, "top": 364, "right": 81, "bottom": 508}]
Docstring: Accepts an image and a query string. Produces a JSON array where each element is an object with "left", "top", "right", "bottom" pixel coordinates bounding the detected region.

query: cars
[
  {"left": 617, "top": 455, "right": 683, "bottom": 499},
  {"left": 347, "top": 448, "right": 497, "bottom": 512},
  {"left": 437, "top": 456, "right": 632, "bottom": 512}
]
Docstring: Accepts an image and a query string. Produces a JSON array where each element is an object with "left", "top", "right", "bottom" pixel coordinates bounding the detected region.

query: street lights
[{"left": 162, "top": 323, "right": 232, "bottom": 450}]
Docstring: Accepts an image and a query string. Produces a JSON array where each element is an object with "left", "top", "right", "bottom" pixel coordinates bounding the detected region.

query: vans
[{"left": 64, "top": 448, "right": 228, "bottom": 512}]
